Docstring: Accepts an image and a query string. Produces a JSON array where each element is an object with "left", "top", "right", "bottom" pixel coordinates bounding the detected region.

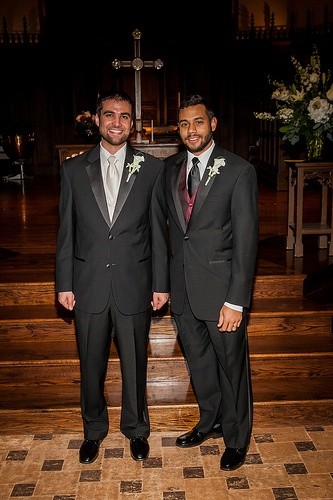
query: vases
[{"left": 306, "top": 131, "right": 324, "bottom": 159}]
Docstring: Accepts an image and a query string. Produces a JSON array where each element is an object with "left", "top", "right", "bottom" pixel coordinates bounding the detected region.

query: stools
[{"left": 281, "top": 160, "right": 333, "bottom": 258}]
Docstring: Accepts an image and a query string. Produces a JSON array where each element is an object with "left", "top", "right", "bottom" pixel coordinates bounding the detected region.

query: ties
[
  {"left": 105, "top": 156, "right": 121, "bottom": 199},
  {"left": 187, "top": 157, "right": 201, "bottom": 200}
]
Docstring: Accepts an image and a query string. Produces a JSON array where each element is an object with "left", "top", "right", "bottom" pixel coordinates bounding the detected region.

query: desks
[{"left": 55, "top": 143, "right": 180, "bottom": 164}]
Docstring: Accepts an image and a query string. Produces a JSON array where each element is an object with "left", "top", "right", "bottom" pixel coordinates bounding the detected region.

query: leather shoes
[
  {"left": 128, "top": 436, "right": 150, "bottom": 461},
  {"left": 79, "top": 437, "right": 104, "bottom": 464},
  {"left": 219, "top": 447, "right": 248, "bottom": 471},
  {"left": 175, "top": 423, "right": 224, "bottom": 448}
]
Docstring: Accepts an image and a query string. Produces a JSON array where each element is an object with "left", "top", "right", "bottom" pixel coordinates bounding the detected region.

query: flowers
[
  {"left": 125, "top": 154, "right": 145, "bottom": 183},
  {"left": 204, "top": 155, "right": 226, "bottom": 186},
  {"left": 255, "top": 49, "right": 333, "bottom": 145}
]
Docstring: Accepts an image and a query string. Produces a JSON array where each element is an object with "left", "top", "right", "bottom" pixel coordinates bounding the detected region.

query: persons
[
  {"left": 57, "top": 95, "right": 170, "bottom": 464},
  {"left": 164, "top": 96, "right": 257, "bottom": 471}
]
